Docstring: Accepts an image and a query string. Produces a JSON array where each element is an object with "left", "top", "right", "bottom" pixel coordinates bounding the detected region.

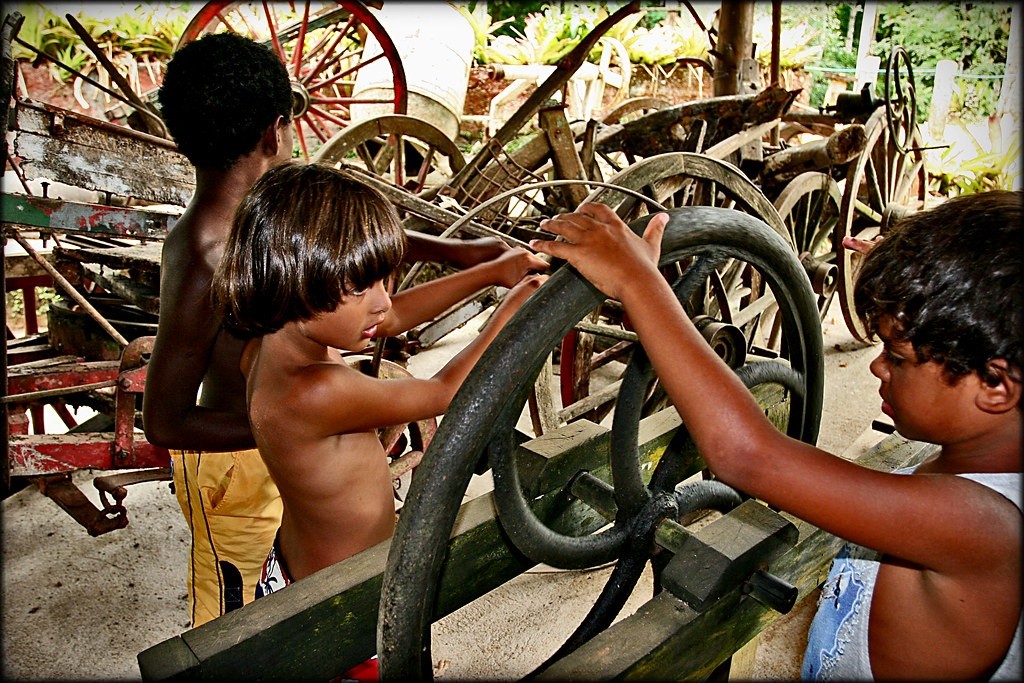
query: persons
[
  {"left": 142, "top": 33, "right": 514, "bottom": 630},
  {"left": 528, "top": 190, "right": 1024, "bottom": 683},
  {"left": 211, "top": 161, "right": 551, "bottom": 683}
]
